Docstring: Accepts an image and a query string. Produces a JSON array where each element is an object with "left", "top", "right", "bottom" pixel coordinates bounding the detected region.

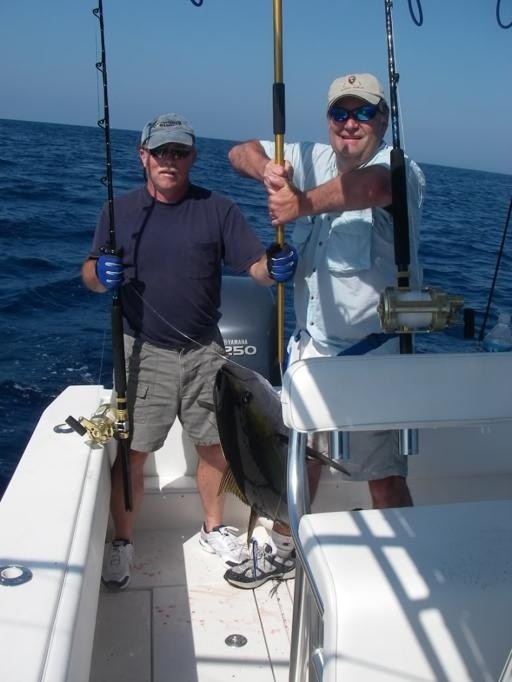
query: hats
[
  {"left": 140, "top": 112, "right": 196, "bottom": 148},
  {"left": 327, "top": 72, "right": 386, "bottom": 110}
]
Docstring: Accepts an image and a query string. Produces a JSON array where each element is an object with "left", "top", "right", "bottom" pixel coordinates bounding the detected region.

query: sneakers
[
  {"left": 199, "top": 521, "right": 250, "bottom": 568},
  {"left": 102, "top": 538, "right": 135, "bottom": 591},
  {"left": 222, "top": 543, "right": 296, "bottom": 590}
]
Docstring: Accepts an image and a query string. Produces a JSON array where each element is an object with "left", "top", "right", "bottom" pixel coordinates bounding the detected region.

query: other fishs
[{"left": 197, "top": 361, "right": 352, "bottom": 550}]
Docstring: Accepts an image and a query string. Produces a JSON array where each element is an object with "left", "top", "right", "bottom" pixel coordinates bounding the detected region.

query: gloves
[
  {"left": 94, "top": 254, "right": 122, "bottom": 291},
  {"left": 265, "top": 242, "right": 298, "bottom": 283}
]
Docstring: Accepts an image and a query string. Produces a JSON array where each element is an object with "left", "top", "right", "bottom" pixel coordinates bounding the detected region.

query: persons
[
  {"left": 222, "top": 71, "right": 424, "bottom": 591},
  {"left": 82, "top": 115, "right": 307, "bottom": 588}
]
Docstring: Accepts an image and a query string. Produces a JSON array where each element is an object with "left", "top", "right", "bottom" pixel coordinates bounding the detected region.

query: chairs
[{"left": 278, "top": 349, "right": 512, "bottom": 682}]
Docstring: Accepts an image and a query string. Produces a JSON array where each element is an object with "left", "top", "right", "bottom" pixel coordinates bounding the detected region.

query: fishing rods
[
  {"left": 66, "top": 0, "right": 134, "bottom": 513},
  {"left": 378, "top": 0, "right": 453, "bottom": 456},
  {"left": 274, "top": 1, "right": 285, "bottom": 388}
]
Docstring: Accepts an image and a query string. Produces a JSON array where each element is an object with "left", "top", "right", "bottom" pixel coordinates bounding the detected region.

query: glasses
[
  {"left": 329, "top": 108, "right": 379, "bottom": 123},
  {"left": 148, "top": 147, "right": 193, "bottom": 161}
]
